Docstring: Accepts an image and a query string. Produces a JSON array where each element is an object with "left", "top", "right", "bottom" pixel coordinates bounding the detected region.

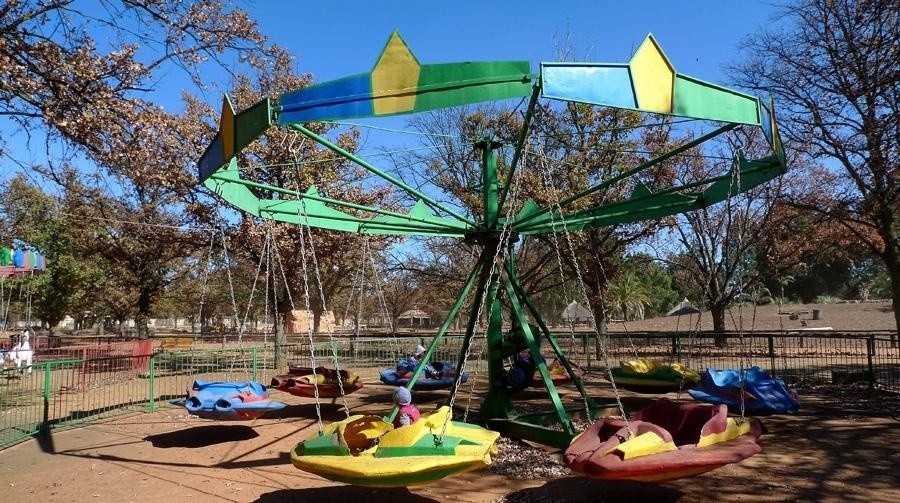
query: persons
[
  {"left": 409, "top": 345, "right": 441, "bottom": 380},
  {"left": 518, "top": 341, "right": 547, "bottom": 367},
  {"left": 369, "top": 385, "right": 419, "bottom": 447}
]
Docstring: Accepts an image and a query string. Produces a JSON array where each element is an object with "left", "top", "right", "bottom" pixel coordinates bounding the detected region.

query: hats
[{"left": 392, "top": 386, "right": 412, "bottom": 406}]
